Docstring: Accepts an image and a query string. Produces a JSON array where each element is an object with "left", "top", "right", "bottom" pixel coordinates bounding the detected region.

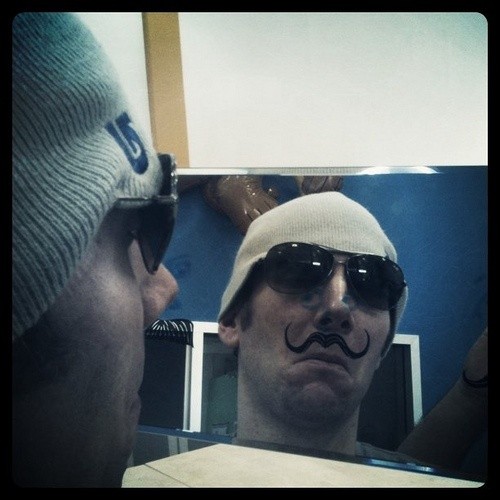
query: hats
[
  {"left": 11, "top": 11, "right": 163, "bottom": 344},
  {"left": 218, "top": 191, "right": 412, "bottom": 354}
]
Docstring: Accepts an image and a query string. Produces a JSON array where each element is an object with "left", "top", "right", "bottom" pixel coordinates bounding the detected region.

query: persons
[
  {"left": 396, "top": 327, "right": 488, "bottom": 469},
  {"left": 218, "top": 190, "right": 452, "bottom": 479},
  {"left": 10, "top": 12, "right": 181, "bottom": 486}
]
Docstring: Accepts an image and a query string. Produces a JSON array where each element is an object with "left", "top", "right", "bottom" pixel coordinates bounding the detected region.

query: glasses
[
  {"left": 112, "top": 149, "right": 177, "bottom": 274},
  {"left": 252, "top": 241, "right": 407, "bottom": 310}
]
